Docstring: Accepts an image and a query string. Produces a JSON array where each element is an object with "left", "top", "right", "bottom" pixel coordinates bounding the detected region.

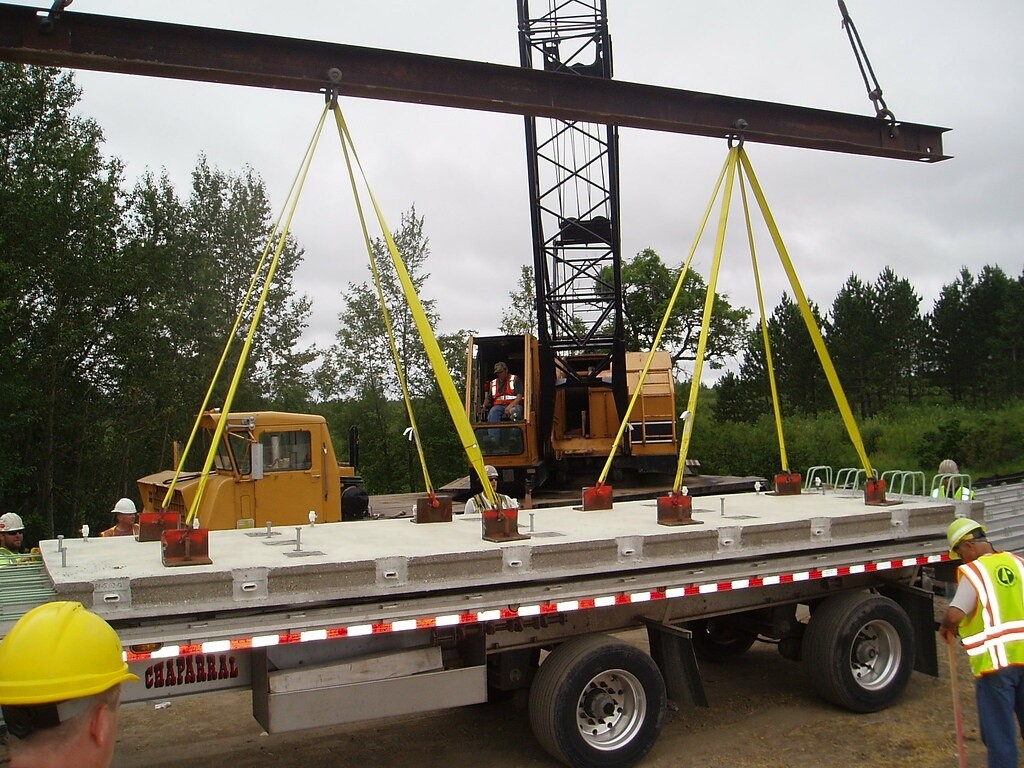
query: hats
[
  {"left": 493, "top": 363, "right": 506, "bottom": 375},
  {"left": 477, "top": 465, "right": 498, "bottom": 481}
]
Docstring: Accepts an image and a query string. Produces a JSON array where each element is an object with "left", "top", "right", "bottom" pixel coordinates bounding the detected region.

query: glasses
[
  {"left": 488, "top": 476, "right": 498, "bottom": 482},
  {"left": 3, "top": 529, "right": 25, "bottom": 535}
]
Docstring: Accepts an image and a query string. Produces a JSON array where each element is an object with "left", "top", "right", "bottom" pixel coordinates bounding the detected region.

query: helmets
[
  {"left": 0, "top": 602, "right": 140, "bottom": 706},
  {"left": 0, "top": 513, "right": 25, "bottom": 532},
  {"left": 938, "top": 460, "right": 959, "bottom": 477},
  {"left": 111, "top": 497, "right": 137, "bottom": 514},
  {"left": 948, "top": 517, "right": 982, "bottom": 559}
]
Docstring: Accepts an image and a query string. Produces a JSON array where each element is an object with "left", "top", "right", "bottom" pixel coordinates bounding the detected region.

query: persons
[
  {"left": 482, "top": 361, "right": 524, "bottom": 446},
  {"left": 100, "top": 498, "right": 140, "bottom": 537},
  {"left": 464, "top": 464, "right": 515, "bottom": 513},
  {"left": 0, "top": 599, "right": 141, "bottom": 768},
  {"left": 937, "top": 515, "right": 1024, "bottom": 768},
  {"left": 932, "top": 459, "right": 976, "bottom": 502},
  {"left": 0, "top": 512, "right": 42, "bottom": 570}
]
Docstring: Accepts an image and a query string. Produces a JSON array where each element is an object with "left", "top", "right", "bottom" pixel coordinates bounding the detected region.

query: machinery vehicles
[{"left": 136, "top": 0, "right": 770, "bottom": 537}]
